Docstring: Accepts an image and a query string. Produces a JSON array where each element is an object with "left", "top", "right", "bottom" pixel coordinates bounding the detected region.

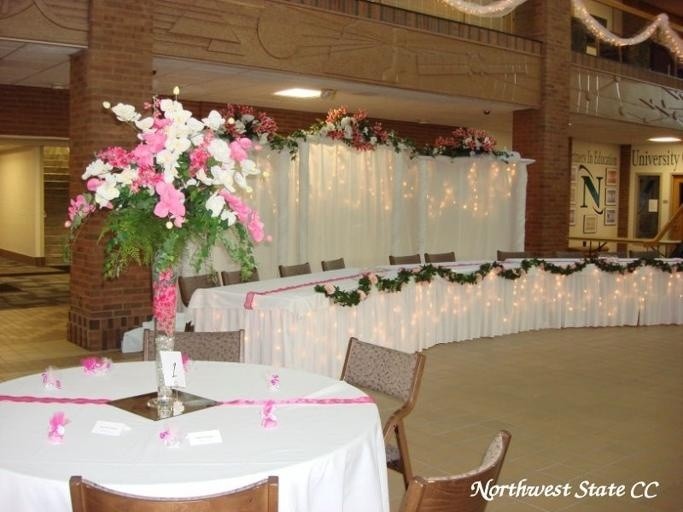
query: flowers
[
  {"left": 419, "top": 124, "right": 521, "bottom": 164},
  {"left": 64, "top": 82, "right": 268, "bottom": 288},
  {"left": 214, "top": 98, "right": 285, "bottom": 151},
  {"left": 288, "top": 106, "right": 416, "bottom": 164}
]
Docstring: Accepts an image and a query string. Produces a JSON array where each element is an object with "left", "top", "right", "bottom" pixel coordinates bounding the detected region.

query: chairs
[
  {"left": 176, "top": 247, "right": 683, "bottom": 376},
  {"left": 0, "top": 327, "right": 511, "bottom": 512}
]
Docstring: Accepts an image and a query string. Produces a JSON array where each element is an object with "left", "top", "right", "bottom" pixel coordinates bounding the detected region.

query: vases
[{"left": 150, "top": 245, "right": 177, "bottom": 344}]
